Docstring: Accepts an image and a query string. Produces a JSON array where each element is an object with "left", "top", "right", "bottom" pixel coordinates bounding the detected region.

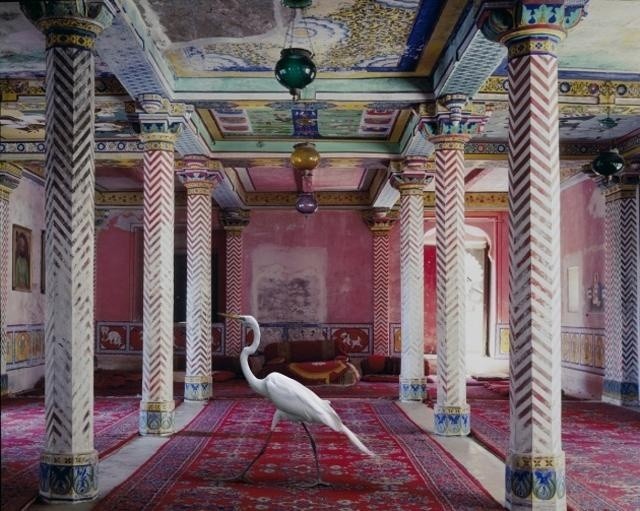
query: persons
[{"left": 15, "top": 232, "right": 28, "bottom": 289}]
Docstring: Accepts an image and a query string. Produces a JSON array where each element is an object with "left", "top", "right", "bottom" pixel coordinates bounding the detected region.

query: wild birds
[{"left": 217, "top": 310, "right": 377, "bottom": 489}]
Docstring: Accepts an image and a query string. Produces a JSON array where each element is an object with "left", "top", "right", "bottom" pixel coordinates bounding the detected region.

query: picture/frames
[{"left": 12, "top": 223, "right": 45, "bottom": 293}]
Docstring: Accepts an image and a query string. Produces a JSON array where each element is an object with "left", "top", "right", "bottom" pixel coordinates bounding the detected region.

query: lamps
[
  {"left": 593, "top": 96, "right": 624, "bottom": 182},
  {"left": 275, "top": 0, "right": 320, "bottom": 218}
]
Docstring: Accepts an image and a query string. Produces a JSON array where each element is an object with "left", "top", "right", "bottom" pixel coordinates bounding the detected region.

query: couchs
[{"left": 259, "top": 342, "right": 430, "bottom": 387}]
